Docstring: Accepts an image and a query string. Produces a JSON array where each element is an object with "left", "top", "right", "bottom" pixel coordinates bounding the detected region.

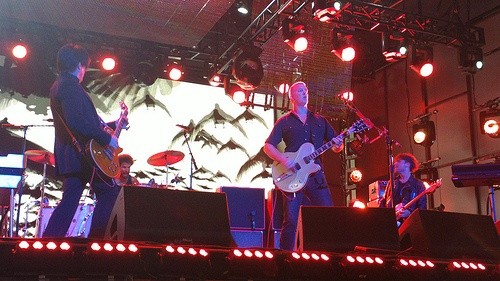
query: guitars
[
  {"left": 83, "top": 100, "right": 128, "bottom": 182},
  {"left": 394, "top": 177, "right": 444, "bottom": 216},
  {"left": 269, "top": 118, "right": 373, "bottom": 194}
]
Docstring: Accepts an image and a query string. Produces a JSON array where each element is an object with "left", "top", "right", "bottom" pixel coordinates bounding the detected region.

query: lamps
[
  {"left": 6, "top": 29, "right": 31, "bottom": 61},
  {"left": 282, "top": 0, "right": 500, "bottom": 190},
  {"left": 223, "top": 46, "right": 264, "bottom": 106},
  {"left": 204, "top": 60, "right": 221, "bottom": 87},
  {"left": 94, "top": 45, "right": 118, "bottom": 73},
  {"left": 162, "top": 48, "right": 185, "bottom": 81}
]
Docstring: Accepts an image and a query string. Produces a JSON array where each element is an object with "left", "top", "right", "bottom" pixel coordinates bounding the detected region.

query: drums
[
  {"left": 35, "top": 204, "right": 58, "bottom": 239},
  {"left": 65, "top": 203, "right": 95, "bottom": 238}
]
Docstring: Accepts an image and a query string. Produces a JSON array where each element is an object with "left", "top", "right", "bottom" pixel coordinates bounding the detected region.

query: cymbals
[
  {"left": 146, "top": 149, "right": 186, "bottom": 166},
  {"left": 24, "top": 149, "right": 57, "bottom": 166}
]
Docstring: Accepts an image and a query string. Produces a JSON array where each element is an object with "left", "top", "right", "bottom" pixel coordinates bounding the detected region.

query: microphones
[
  {"left": 177, "top": 124, "right": 189, "bottom": 131},
  {"left": 251, "top": 211, "right": 256, "bottom": 230}
]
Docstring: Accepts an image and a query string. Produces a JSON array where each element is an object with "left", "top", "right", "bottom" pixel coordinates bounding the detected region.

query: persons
[
  {"left": 117, "top": 153, "right": 138, "bottom": 184},
  {"left": 379, "top": 153, "right": 427, "bottom": 227},
  {"left": 263, "top": 81, "right": 343, "bottom": 251},
  {"left": 42, "top": 44, "right": 119, "bottom": 237}
]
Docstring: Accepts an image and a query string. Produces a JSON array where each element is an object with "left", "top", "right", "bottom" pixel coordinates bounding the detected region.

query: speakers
[
  {"left": 230, "top": 230, "right": 263, "bottom": 248},
  {"left": 41, "top": 203, "right": 94, "bottom": 238},
  {"left": 293, "top": 205, "right": 400, "bottom": 253},
  {"left": 216, "top": 186, "right": 266, "bottom": 231},
  {"left": 103, "top": 184, "right": 231, "bottom": 246},
  {"left": 398, "top": 208, "right": 500, "bottom": 262},
  {"left": 270, "top": 231, "right": 281, "bottom": 249},
  {"left": 268, "top": 189, "right": 284, "bottom": 231}
]
